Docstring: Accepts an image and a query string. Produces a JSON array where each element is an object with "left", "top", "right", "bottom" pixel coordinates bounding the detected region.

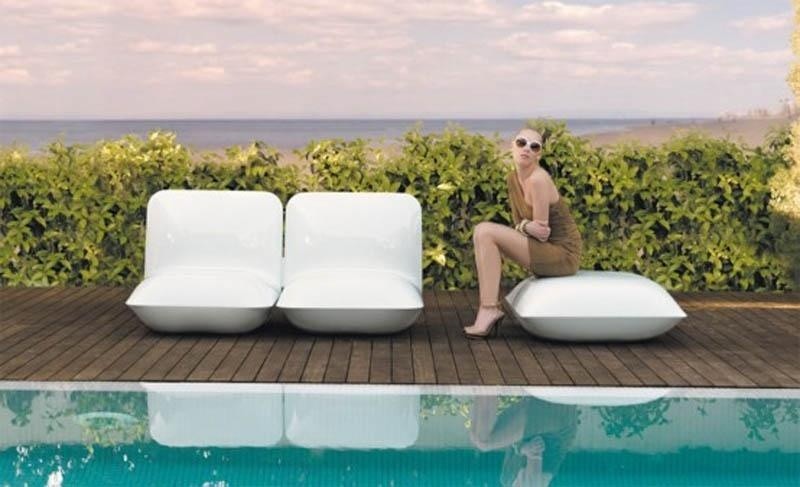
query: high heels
[{"left": 462, "top": 303, "right": 505, "bottom": 340}]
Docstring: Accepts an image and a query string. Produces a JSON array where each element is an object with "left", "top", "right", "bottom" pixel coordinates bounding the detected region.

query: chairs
[
  {"left": 124, "top": 189, "right": 283, "bottom": 336},
  {"left": 148, "top": 392, "right": 285, "bottom": 447},
  {"left": 282, "top": 394, "right": 420, "bottom": 450},
  {"left": 276, "top": 191, "right": 423, "bottom": 335}
]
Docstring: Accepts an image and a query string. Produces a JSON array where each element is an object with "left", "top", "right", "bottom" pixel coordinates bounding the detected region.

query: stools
[
  {"left": 504, "top": 270, "right": 688, "bottom": 343},
  {"left": 533, "top": 396, "right": 660, "bottom": 407}
]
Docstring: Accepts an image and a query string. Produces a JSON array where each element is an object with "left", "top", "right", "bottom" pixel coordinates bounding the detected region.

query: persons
[
  {"left": 463, "top": 126, "right": 583, "bottom": 338},
  {"left": 471, "top": 397, "right": 580, "bottom": 487}
]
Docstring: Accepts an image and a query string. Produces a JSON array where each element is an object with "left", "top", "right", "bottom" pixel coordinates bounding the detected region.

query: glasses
[{"left": 512, "top": 138, "right": 540, "bottom": 153}]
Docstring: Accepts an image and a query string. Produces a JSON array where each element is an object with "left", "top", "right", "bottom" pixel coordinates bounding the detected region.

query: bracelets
[{"left": 520, "top": 219, "right": 531, "bottom": 236}]
